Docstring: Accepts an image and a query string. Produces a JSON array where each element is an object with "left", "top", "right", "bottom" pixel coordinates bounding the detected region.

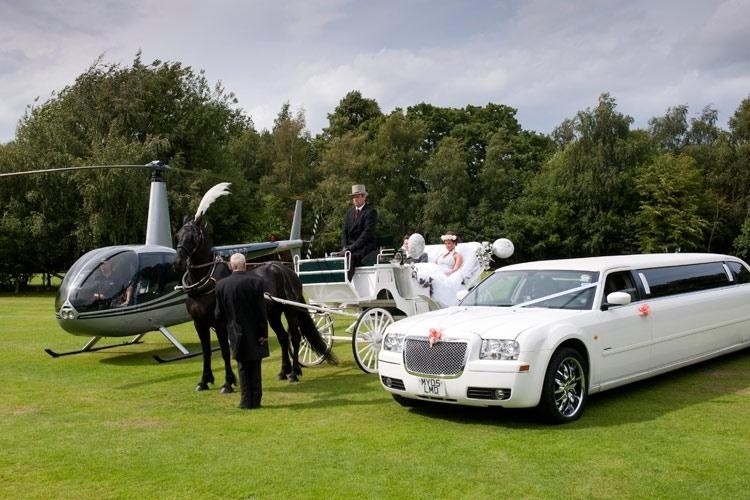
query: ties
[{"left": 354, "top": 209, "right": 360, "bottom": 222}]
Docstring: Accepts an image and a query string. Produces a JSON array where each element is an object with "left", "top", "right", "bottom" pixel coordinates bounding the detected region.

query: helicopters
[{"left": 0, "top": 159, "right": 313, "bottom": 363}]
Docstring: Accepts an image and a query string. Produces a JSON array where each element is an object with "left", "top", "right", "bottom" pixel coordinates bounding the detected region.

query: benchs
[{"left": 411, "top": 241, "right": 486, "bottom": 291}]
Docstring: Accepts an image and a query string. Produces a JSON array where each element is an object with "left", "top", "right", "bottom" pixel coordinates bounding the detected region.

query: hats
[{"left": 348, "top": 184, "right": 369, "bottom": 196}]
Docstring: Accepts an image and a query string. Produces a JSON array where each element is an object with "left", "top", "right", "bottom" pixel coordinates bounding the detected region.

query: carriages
[{"left": 174, "top": 211, "right": 515, "bottom": 393}]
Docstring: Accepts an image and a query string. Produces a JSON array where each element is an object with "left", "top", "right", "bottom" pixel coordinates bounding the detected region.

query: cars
[{"left": 377, "top": 254, "right": 746, "bottom": 422}]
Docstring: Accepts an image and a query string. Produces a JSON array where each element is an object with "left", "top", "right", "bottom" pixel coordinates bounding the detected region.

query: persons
[
  {"left": 212, "top": 251, "right": 270, "bottom": 409},
  {"left": 387, "top": 235, "right": 429, "bottom": 264},
  {"left": 416, "top": 231, "right": 463, "bottom": 309},
  {"left": 92, "top": 259, "right": 133, "bottom": 310},
  {"left": 331, "top": 184, "right": 379, "bottom": 282}
]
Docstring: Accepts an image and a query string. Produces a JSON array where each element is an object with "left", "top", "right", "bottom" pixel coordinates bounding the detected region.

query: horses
[{"left": 174, "top": 211, "right": 339, "bottom": 394}]
